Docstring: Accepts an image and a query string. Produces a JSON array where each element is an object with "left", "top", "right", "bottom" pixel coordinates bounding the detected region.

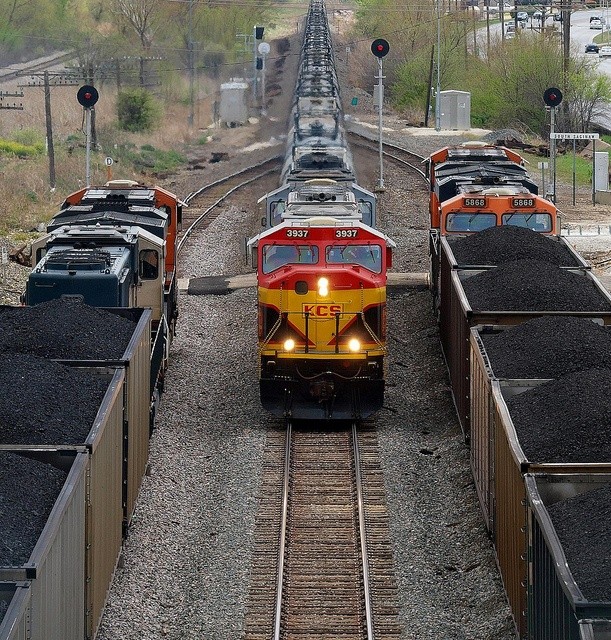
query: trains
[
  {"left": 419, "top": 139, "right": 611, "bottom": 637},
  {"left": 247, "top": 0, "right": 398, "bottom": 425},
  {"left": 0, "top": 181, "right": 188, "bottom": 639}
]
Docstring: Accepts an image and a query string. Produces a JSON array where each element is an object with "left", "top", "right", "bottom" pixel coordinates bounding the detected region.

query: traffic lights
[
  {"left": 541, "top": 87, "right": 563, "bottom": 202},
  {"left": 253, "top": 23, "right": 264, "bottom": 106},
  {"left": 370, "top": 38, "right": 390, "bottom": 192},
  {"left": 76, "top": 85, "right": 99, "bottom": 186}
]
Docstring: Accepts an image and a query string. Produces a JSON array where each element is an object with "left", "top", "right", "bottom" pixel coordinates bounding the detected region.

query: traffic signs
[{"left": 104, "top": 157, "right": 113, "bottom": 181}]
